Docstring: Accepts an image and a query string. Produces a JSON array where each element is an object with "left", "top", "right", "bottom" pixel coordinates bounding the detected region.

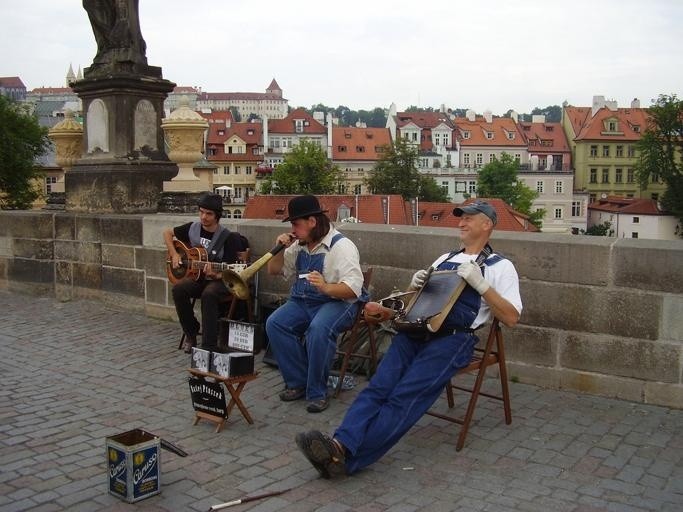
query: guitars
[{"left": 165, "top": 240, "right": 247, "bottom": 285}]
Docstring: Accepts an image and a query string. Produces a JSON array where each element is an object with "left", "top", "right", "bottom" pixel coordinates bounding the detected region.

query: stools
[{"left": 188, "top": 368, "right": 258, "bottom": 432}]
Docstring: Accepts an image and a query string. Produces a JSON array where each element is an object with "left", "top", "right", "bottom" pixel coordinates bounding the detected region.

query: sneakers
[
  {"left": 278, "top": 386, "right": 305, "bottom": 401},
  {"left": 306, "top": 398, "right": 328, "bottom": 412},
  {"left": 180, "top": 332, "right": 196, "bottom": 354},
  {"left": 294, "top": 429, "right": 346, "bottom": 479}
]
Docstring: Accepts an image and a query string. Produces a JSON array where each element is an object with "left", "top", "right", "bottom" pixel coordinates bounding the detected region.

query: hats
[
  {"left": 451, "top": 198, "right": 497, "bottom": 227},
  {"left": 281, "top": 195, "right": 328, "bottom": 222},
  {"left": 189, "top": 193, "right": 223, "bottom": 211}
]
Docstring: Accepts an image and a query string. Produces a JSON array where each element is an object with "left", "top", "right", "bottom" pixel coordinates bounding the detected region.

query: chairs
[
  {"left": 178, "top": 247, "right": 253, "bottom": 352},
  {"left": 329, "top": 267, "right": 379, "bottom": 398},
  {"left": 425, "top": 317, "right": 512, "bottom": 451}
]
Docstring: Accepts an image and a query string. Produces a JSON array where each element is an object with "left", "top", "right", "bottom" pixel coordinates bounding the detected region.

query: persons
[
  {"left": 163, "top": 192, "right": 240, "bottom": 354},
  {"left": 268, "top": 195, "right": 364, "bottom": 414},
  {"left": 295, "top": 200, "right": 524, "bottom": 480}
]
